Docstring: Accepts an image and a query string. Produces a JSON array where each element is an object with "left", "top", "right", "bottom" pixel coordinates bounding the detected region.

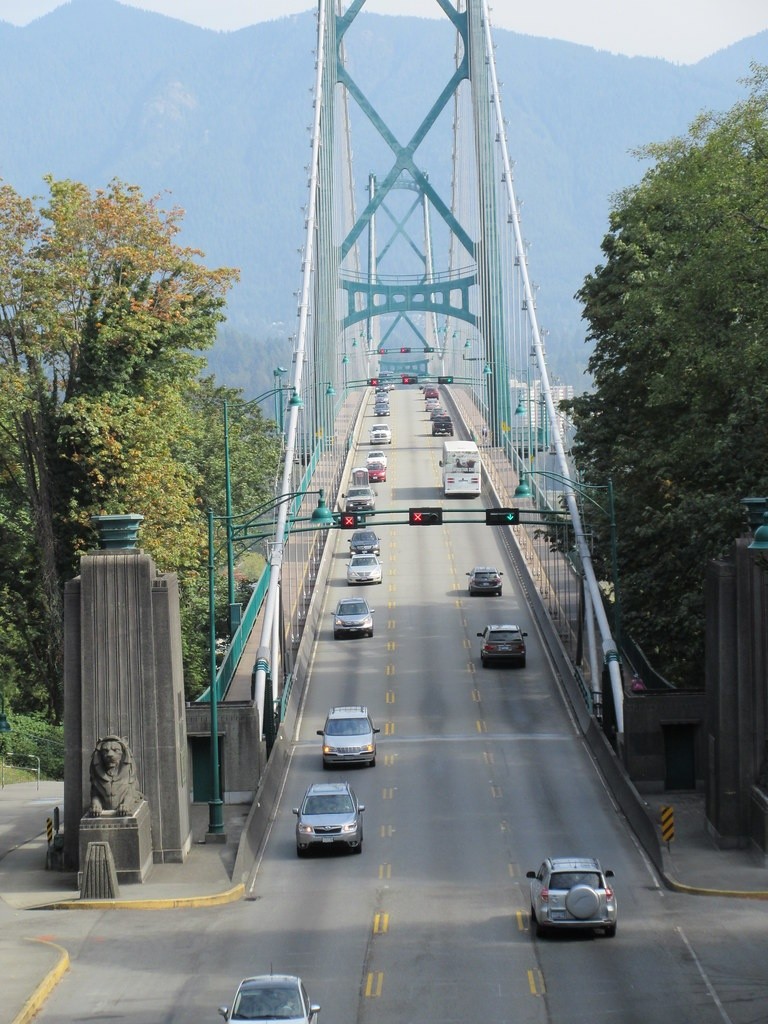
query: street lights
[
  {"left": 512, "top": 469, "right": 624, "bottom": 690},
  {"left": 273, "top": 371, "right": 337, "bottom": 437},
  {"left": 223, "top": 387, "right": 303, "bottom": 605},
  {"left": 483, "top": 361, "right": 534, "bottom": 499},
  {"left": 514, "top": 398, "right": 583, "bottom": 509},
  {"left": 464, "top": 337, "right": 487, "bottom": 422},
  {"left": 208, "top": 489, "right": 334, "bottom": 844}
]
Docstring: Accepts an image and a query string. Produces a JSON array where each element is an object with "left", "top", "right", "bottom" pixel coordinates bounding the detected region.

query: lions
[{"left": 89, "top": 735, "right": 145, "bottom": 817}]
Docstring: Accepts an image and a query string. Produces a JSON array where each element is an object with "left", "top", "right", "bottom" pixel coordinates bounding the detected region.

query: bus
[{"left": 439, "top": 440, "right": 481, "bottom": 497}]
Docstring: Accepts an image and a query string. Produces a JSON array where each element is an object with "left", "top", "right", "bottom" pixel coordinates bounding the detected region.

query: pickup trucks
[
  {"left": 342, "top": 482, "right": 378, "bottom": 516},
  {"left": 431, "top": 415, "right": 454, "bottom": 436}
]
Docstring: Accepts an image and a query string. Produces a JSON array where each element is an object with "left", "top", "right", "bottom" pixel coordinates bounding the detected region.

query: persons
[
  {"left": 482, "top": 426, "right": 488, "bottom": 441},
  {"left": 632, "top": 674, "right": 645, "bottom": 689}
]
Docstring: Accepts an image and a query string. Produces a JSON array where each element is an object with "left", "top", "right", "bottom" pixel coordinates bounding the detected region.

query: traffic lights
[
  {"left": 424, "top": 348, "right": 434, "bottom": 353},
  {"left": 438, "top": 376, "right": 453, "bottom": 385},
  {"left": 401, "top": 348, "right": 411, "bottom": 353},
  {"left": 340, "top": 513, "right": 358, "bottom": 530},
  {"left": 370, "top": 378, "right": 378, "bottom": 386},
  {"left": 410, "top": 508, "right": 443, "bottom": 526},
  {"left": 485, "top": 508, "right": 519, "bottom": 526},
  {"left": 379, "top": 349, "right": 384, "bottom": 354},
  {"left": 401, "top": 377, "right": 419, "bottom": 384}
]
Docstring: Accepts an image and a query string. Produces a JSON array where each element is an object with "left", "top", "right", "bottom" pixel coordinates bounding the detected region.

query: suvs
[
  {"left": 477, "top": 624, "right": 528, "bottom": 667},
  {"left": 526, "top": 857, "right": 618, "bottom": 938},
  {"left": 292, "top": 780, "right": 366, "bottom": 857},
  {"left": 368, "top": 424, "right": 391, "bottom": 445},
  {"left": 317, "top": 706, "right": 379, "bottom": 771}
]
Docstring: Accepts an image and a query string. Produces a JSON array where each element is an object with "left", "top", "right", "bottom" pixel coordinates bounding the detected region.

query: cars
[
  {"left": 217, "top": 973, "right": 320, "bottom": 1024},
  {"left": 419, "top": 384, "right": 447, "bottom": 420},
  {"left": 330, "top": 598, "right": 375, "bottom": 640},
  {"left": 347, "top": 531, "right": 381, "bottom": 558},
  {"left": 364, "top": 451, "right": 388, "bottom": 482},
  {"left": 466, "top": 567, "right": 503, "bottom": 596},
  {"left": 345, "top": 554, "right": 384, "bottom": 586},
  {"left": 372, "top": 371, "right": 395, "bottom": 416}
]
